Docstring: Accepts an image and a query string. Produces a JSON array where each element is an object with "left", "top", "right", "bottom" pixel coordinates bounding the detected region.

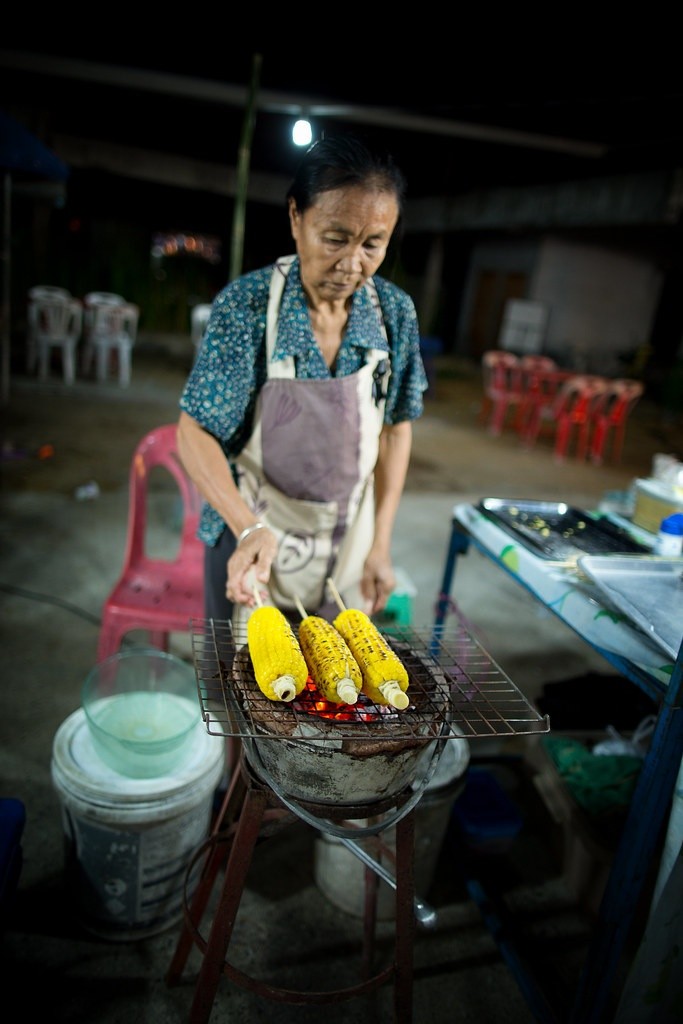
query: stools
[{"left": 170, "top": 754, "right": 419, "bottom": 1024}]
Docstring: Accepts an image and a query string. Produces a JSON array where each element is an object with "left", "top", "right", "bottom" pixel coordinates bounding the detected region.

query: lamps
[{"left": 292, "top": 111, "right": 313, "bottom": 147}]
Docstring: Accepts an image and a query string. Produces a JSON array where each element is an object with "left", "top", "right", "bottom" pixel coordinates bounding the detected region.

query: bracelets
[{"left": 236, "top": 521, "right": 269, "bottom": 548}]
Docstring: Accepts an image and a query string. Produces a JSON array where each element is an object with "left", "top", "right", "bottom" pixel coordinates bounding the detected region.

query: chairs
[
  {"left": 84, "top": 292, "right": 137, "bottom": 386},
  {"left": 97, "top": 424, "right": 205, "bottom": 695},
  {"left": 27, "top": 285, "right": 80, "bottom": 387},
  {"left": 482, "top": 352, "right": 641, "bottom": 464}
]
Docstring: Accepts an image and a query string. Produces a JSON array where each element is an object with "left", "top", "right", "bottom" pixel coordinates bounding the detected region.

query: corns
[{"left": 246, "top": 604, "right": 410, "bottom": 710}]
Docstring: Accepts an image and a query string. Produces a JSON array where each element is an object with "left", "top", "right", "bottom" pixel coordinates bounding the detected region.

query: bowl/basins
[
  {"left": 81, "top": 644, "right": 207, "bottom": 781},
  {"left": 630, "top": 479, "right": 682, "bottom": 535},
  {"left": 455, "top": 769, "right": 521, "bottom": 852}
]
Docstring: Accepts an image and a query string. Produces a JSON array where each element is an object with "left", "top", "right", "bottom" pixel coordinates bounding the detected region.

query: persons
[{"left": 174, "top": 134, "right": 428, "bottom": 896}]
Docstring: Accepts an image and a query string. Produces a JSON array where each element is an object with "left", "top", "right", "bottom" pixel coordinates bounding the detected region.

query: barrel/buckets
[
  {"left": 52, "top": 693, "right": 227, "bottom": 945},
  {"left": 312, "top": 719, "right": 471, "bottom": 921}
]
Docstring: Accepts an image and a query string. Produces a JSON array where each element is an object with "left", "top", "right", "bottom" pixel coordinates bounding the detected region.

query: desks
[{"left": 432, "top": 505, "right": 682, "bottom": 1023}]
